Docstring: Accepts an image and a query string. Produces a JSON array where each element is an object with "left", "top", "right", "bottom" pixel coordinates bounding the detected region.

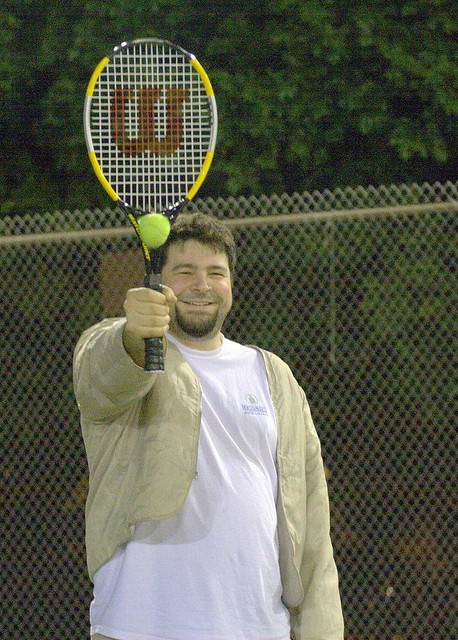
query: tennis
[{"left": 137, "top": 213, "right": 171, "bottom": 249}]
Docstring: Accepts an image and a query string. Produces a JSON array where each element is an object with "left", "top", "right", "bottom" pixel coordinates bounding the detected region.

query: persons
[{"left": 72, "top": 212, "right": 345, "bottom": 639}]
